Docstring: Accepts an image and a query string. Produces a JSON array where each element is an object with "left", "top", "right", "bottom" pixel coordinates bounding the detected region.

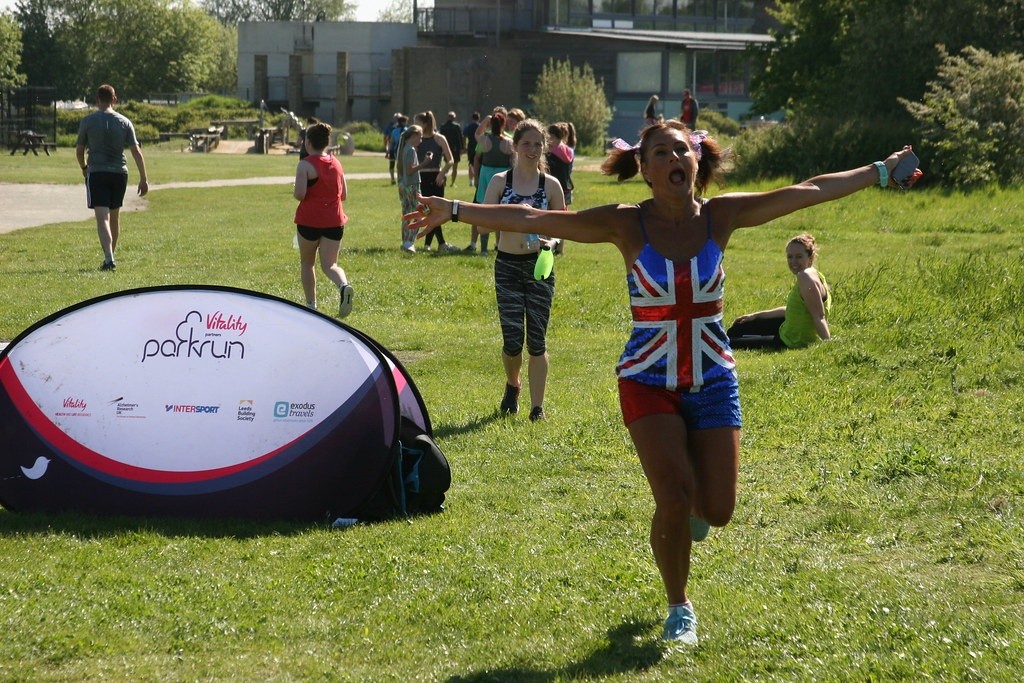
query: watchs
[{"left": 554, "top": 239, "right": 560, "bottom": 250}]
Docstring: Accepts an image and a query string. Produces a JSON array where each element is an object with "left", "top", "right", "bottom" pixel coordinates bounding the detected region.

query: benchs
[{"left": 7, "top": 143, "right": 56, "bottom": 156}]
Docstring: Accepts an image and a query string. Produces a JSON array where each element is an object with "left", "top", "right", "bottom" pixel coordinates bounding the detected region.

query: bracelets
[
  {"left": 82, "top": 165, "right": 87, "bottom": 170},
  {"left": 873, "top": 161, "right": 888, "bottom": 187},
  {"left": 451, "top": 199, "right": 460, "bottom": 223}
]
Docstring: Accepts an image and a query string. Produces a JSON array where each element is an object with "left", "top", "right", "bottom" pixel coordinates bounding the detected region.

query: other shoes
[
  {"left": 401, "top": 244, "right": 417, "bottom": 254},
  {"left": 100, "top": 260, "right": 116, "bottom": 271},
  {"left": 463, "top": 246, "right": 477, "bottom": 252},
  {"left": 439, "top": 243, "right": 462, "bottom": 253},
  {"left": 339, "top": 285, "right": 354, "bottom": 318},
  {"left": 424, "top": 245, "right": 431, "bottom": 252},
  {"left": 480, "top": 252, "right": 489, "bottom": 256}
]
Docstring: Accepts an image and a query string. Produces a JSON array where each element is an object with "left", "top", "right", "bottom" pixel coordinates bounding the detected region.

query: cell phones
[{"left": 891, "top": 145, "right": 920, "bottom": 190}]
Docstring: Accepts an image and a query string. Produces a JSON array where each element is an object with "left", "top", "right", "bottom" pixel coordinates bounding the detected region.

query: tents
[{"left": 1, "top": 284, "right": 452, "bottom": 520}]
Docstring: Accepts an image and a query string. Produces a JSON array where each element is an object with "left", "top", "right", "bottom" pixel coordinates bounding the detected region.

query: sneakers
[
  {"left": 500, "top": 378, "right": 522, "bottom": 415},
  {"left": 689, "top": 508, "right": 710, "bottom": 541},
  {"left": 529, "top": 405, "right": 547, "bottom": 425},
  {"left": 662, "top": 608, "right": 698, "bottom": 649}
]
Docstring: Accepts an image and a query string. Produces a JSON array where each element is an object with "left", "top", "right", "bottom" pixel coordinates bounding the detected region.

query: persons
[
  {"left": 644, "top": 95, "right": 659, "bottom": 125},
  {"left": 383, "top": 112, "right": 460, "bottom": 252},
  {"left": 538, "top": 121, "right": 577, "bottom": 255},
  {"left": 297, "top": 118, "right": 318, "bottom": 160},
  {"left": 476, "top": 121, "right": 565, "bottom": 421},
  {"left": 401, "top": 121, "right": 923, "bottom": 648},
  {"left": 681, "top": 89, "right": 699, "bottom": 131},
  {"left": 76, "top": 85, "right": 149, "bottom": 270},
  {"left": 725, "top": 234, "right": 832, "bottom": 349},
  {"left": 293, "top": 124, "right": 354, "bottom": 318},
  {"left": 440, "top": 107, "right": 525, "bottom": 254}
]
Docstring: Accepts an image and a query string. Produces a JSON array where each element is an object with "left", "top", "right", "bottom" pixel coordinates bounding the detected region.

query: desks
[{"left": 13, "top": 134, "right": 48, "bottom": 157}]
